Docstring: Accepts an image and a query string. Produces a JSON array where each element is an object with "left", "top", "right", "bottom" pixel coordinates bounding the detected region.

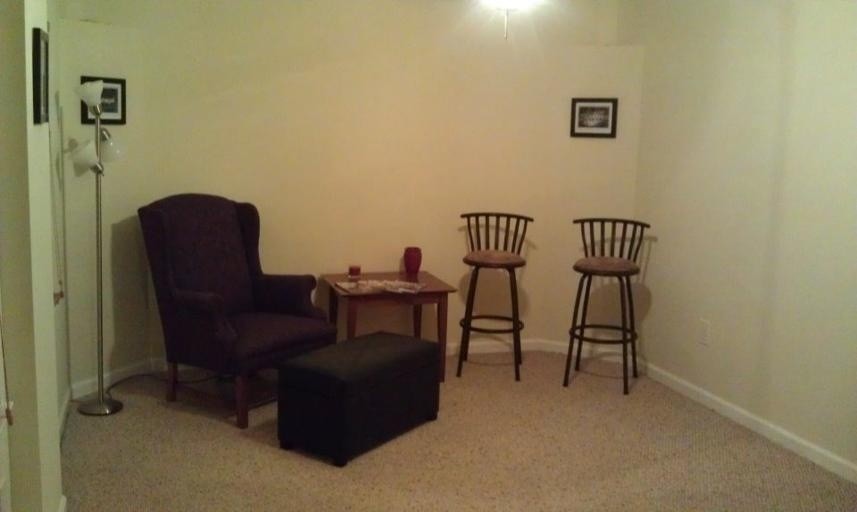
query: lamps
[{"left": 72, "top": 80, "right": 125, "bottom": 416}]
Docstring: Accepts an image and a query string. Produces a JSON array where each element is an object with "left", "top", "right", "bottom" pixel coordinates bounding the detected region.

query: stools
[{"left": 277, "top": 333, "right": 440, "bottom": 466}]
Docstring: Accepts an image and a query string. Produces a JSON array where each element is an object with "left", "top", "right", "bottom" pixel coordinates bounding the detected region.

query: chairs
[
  {"left": 563, "top": 218, "right": 650, "bottom": 395},
  {"left": 138, "top": 194, "right": 337, "bottom": 429},
  {"left": 457, "top": 212, "right": 533, "bottom": 380}
]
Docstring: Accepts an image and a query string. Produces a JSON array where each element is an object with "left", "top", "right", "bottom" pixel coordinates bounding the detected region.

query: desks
[{"left": 322, "top": 273, "right": 456, "bottom": 382}]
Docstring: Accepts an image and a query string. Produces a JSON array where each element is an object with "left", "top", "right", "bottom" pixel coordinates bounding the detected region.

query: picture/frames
[
  {"left": 32, "top": 28, "right": 49, "bottom": 124},
  {"left": 80, "top": 76, "right": 126, "bottom": 126},
  {"left": 571, "top": 97, "right": 617, "bottom": 138}
]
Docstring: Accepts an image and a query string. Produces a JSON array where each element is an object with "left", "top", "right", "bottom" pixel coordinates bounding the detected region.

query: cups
[
  {"left": 349, "top": 263, "right": 361, "bottom": 278},
  {"left": 405, "top": 247, "right": 422, "bottom": 274}
]
